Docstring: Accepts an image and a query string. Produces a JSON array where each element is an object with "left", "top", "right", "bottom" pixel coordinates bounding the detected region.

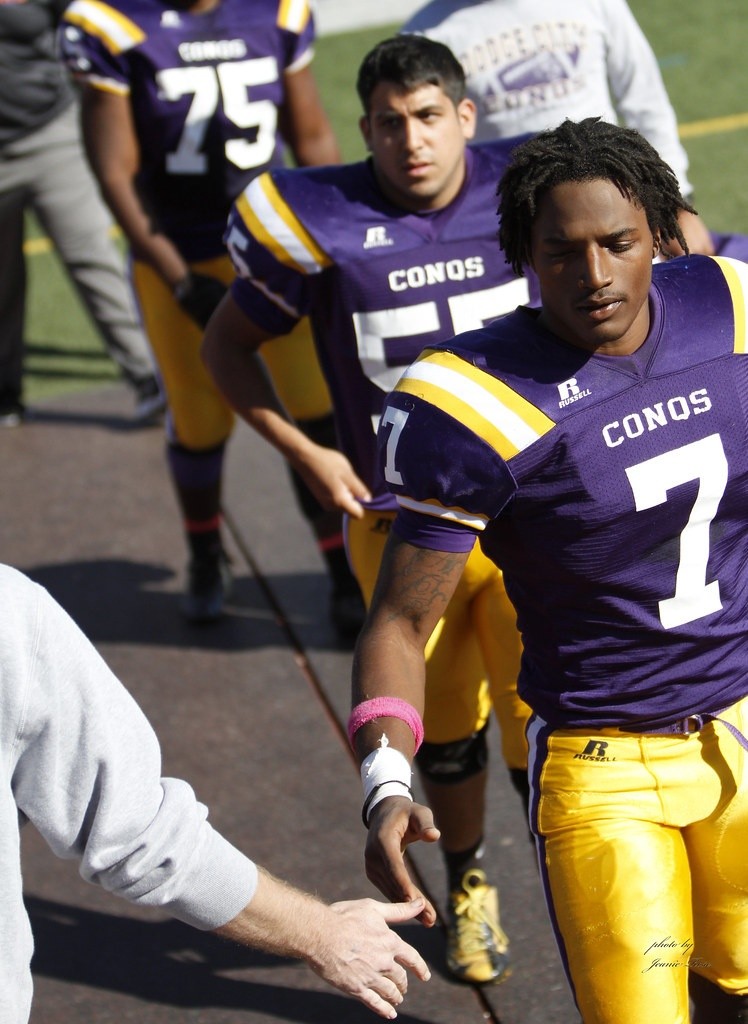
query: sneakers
[{"left": 447, "top": 867, "right": 509, "bottom": 980}]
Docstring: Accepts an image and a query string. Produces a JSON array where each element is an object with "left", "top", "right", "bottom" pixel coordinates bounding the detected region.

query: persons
[{"left": 0, "top": 1, "right": 748, "bottom": 1024}]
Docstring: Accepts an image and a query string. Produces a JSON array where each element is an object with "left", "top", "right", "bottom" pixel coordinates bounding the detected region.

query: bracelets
[
  {"left": 366, "top": 781, "right": 415, "bottom": 828},
  {"left": 348, "top": 696, "right": 424, "bottom": 755},
  {"left": 356, "top": 746, "right": 412, "bottom": 825}
]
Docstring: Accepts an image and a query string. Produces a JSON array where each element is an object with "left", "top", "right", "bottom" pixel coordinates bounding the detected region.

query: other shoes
[
  {"left": 183, "top": 552, "right": 230, "bottom": 628},
  {"left": 1, "top": 380, "right": 23, "bottom": 425},
  {"left": 328, "top": 569, "right": 366, "bottom": 653},
  {"left": 137, "top": 373, "right": 171, "bottom": 417}
]
